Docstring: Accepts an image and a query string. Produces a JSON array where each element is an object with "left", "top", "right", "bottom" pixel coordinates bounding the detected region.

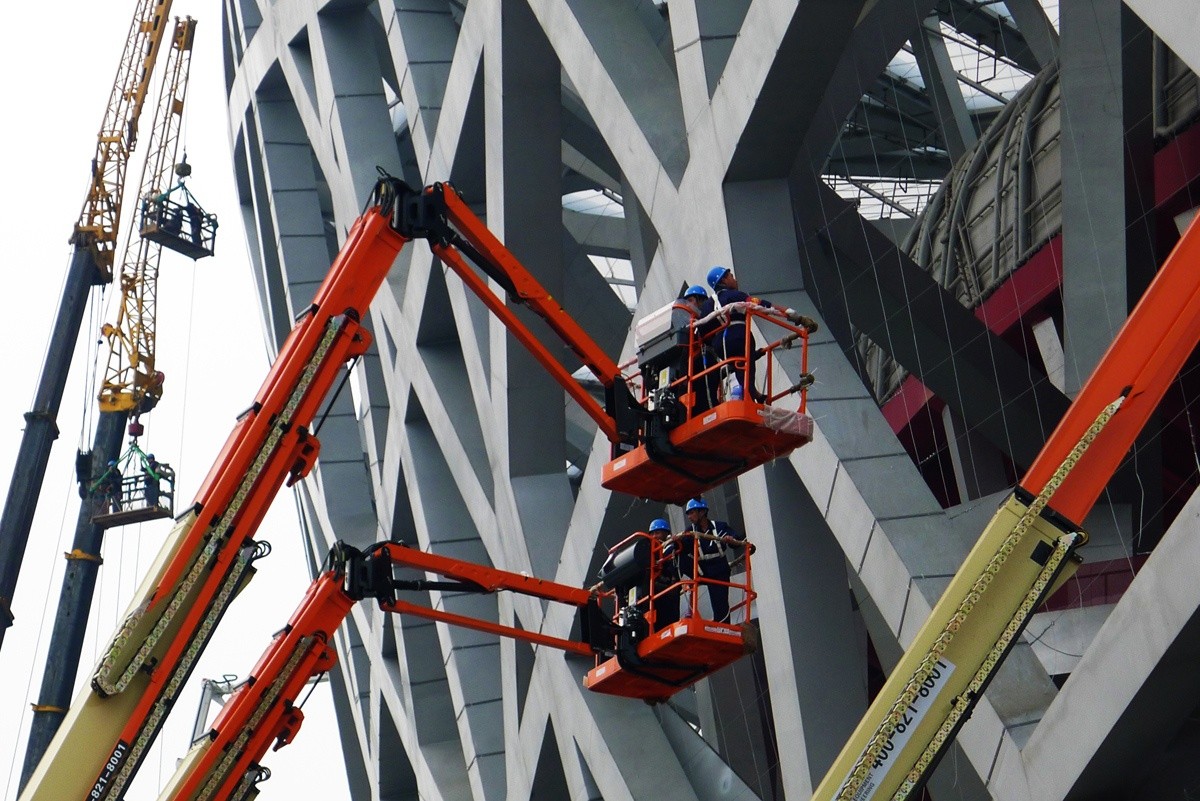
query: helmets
[
  {"left": 649, "top": 518, "right": 671, "bottom": 533},
  {"left": 684, "top": 285, "right": 708, "bottom": 300},
  {"left": 107, "top": 460, "right": 117, "bottom": 468},
  {"left": 146, "top": 453, "right": 155, "bottom": 459},
  {"left": 707, "top": 266, "right": 730, "bottom": 290},
  {"left": 685, "top": 498, "right": 709, "bottom": 514}
]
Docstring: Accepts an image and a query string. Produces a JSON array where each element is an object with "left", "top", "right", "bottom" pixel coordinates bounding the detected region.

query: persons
[
  {"left": 107, "top": 455, "right": 162, "bottom": 513},
  {"left": 684, "top": 267, "right": 817, "bottom": 418},
  {"left": 634, "top": 498, "right": 743, "bottom": 633},
  {"left": 180, "top": 202, "right": 204, "bottom": 245}
]
